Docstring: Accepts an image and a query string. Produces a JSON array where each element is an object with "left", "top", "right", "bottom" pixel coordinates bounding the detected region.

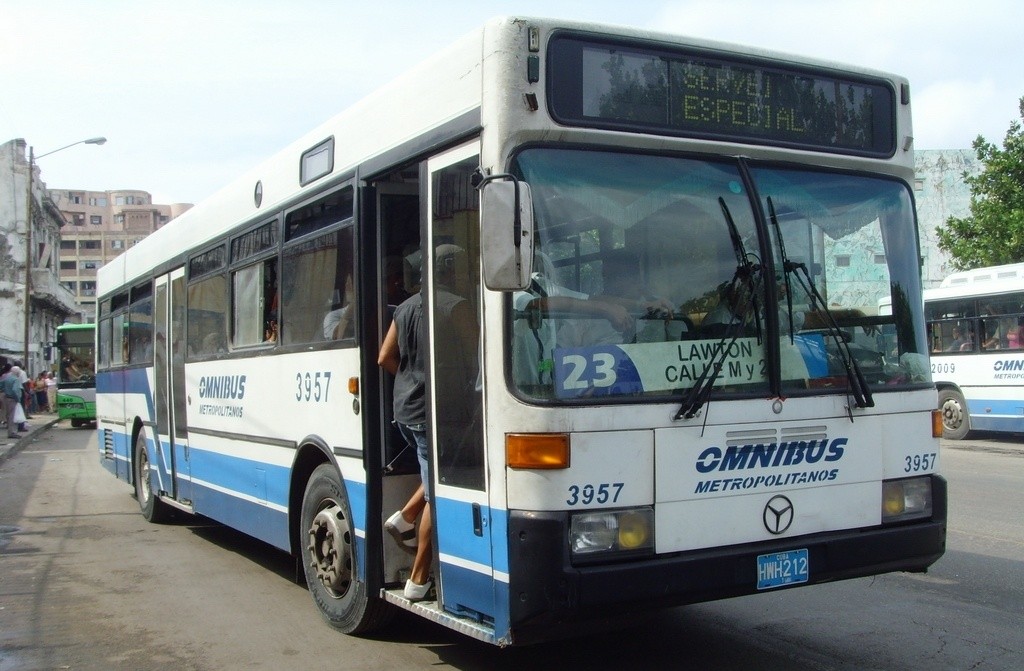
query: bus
[
  {"left": 42, "top": 321, "right": 95, "bottom": 428},
  {"left": 94, "top": 8, "right": 948, "bottom": 649},
  {"left": 877, "top": 261, "right": 1024, "bottom": 439}
]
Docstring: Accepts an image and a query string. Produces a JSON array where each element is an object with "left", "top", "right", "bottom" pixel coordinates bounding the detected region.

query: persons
[
  {"left": 0, "top": 361, "right": 58, "bottom": 439},
  {"left": 323, "top": 270, "right": 399, "bottom": 341},
  {"left": 932, "top": 319, "right": 1024, "bottom": 352},
  {"left": 378, "top": 245, "right": 483, "bottom": 602},
  {"left": 511, "top": 247, "right": 804, "bottom": 387},
  {"left": 264, "top": 320, "right": 279, "bottom": 342},
  {"left": 61, "top": 352, "right": 83, "bottom": 382}
]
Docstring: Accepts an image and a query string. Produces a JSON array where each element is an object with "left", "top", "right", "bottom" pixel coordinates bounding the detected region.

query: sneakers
[
  {"left": 404, "top": 576, "right": 437, "bottom": 602},
  {"left": 384, "top": 511, "right": 418, "bottom": 556}
]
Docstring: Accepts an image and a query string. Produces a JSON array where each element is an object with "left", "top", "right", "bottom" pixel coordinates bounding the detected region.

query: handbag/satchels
[{"left": 13, "top": 402, "right": 29, "bottom": 424}]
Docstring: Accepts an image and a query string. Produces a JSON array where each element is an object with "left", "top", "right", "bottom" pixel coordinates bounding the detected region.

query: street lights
[{"left": 22, "top": 136, "right": 106, "bottom": 370}]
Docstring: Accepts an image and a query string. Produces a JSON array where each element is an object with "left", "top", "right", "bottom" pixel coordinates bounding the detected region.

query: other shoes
[
  {"left": 7, "top": 431, "right": 21, "bottom": 439},
  {"left": 17, "top": 428, "right": 29, "bottom": 432}
]
